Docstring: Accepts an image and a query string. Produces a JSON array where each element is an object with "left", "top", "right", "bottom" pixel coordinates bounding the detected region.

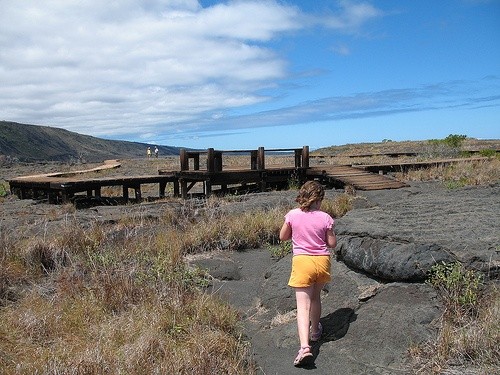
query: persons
[
  {"left": 146, "top": 146, "right": 152, "bottom": 159},
  {"left": 152, "top": 145, "right": 159, "bottom": 158},
  {"left": 278, "top": 180, "right": 338, "bottom": 368}
]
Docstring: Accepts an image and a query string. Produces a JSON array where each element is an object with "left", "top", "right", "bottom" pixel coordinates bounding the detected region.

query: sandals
[
  {"left": 294, "top": 345, "right": 313, "bottom": 365},
  {"left": 310, "top": 322, "right": 322, "bottom": 342}
]
